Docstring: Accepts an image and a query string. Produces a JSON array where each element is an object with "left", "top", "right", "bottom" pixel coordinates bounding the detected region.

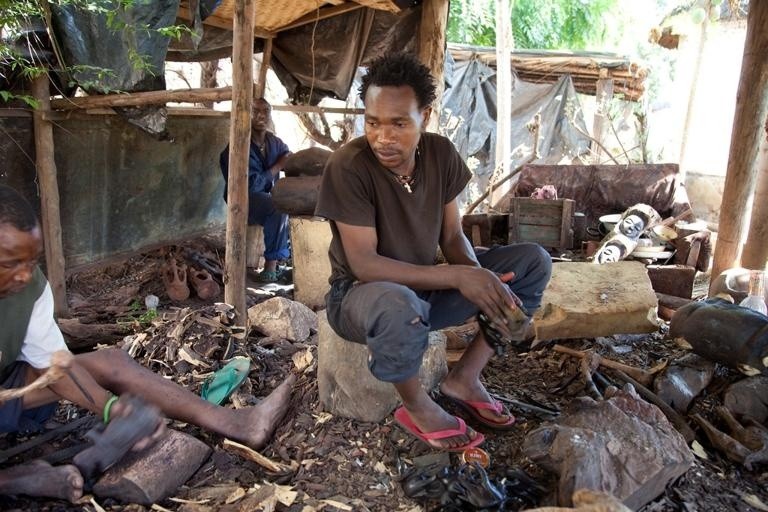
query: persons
[
  {"left": 598, "top": 239, "right": 626, "bottom": 264},
  {"left": 619, "top": 209, "right": 648, "bottom": 239},
  {"left": 219, "top": 97, "right": 295, "bottom": 284},
  {"left": 1, "top": 184, "right": 296, "bottom": 505},
  {"left": 314, "top": 54, "right": 552, "bottom": 453}
]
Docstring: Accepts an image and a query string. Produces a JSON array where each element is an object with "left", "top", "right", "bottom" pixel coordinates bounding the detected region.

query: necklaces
[
  {"left": 393, "top": 145, "right": 422, "bottom": 194},
  {"left": 257, "top": 141, "right": 266, "bottom": 151}
]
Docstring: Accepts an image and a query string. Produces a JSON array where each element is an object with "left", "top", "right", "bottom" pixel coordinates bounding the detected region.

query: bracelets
[{"left": 102, "top": 396, "right": 119, "bottom": 425}]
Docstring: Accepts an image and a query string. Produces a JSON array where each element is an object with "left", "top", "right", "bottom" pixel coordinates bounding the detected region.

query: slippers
[
  {"left": 439, "top": 389, "right": 516, "bottom": 429},
  {"left": 394, "top": 406, "right": 485, "bottom": 453},
  {"left": 201, "top": 358, "right": 252, "bottom": 406},
  {"left": 259, "top": 271, "right": 279, "bottom": 282}
]
